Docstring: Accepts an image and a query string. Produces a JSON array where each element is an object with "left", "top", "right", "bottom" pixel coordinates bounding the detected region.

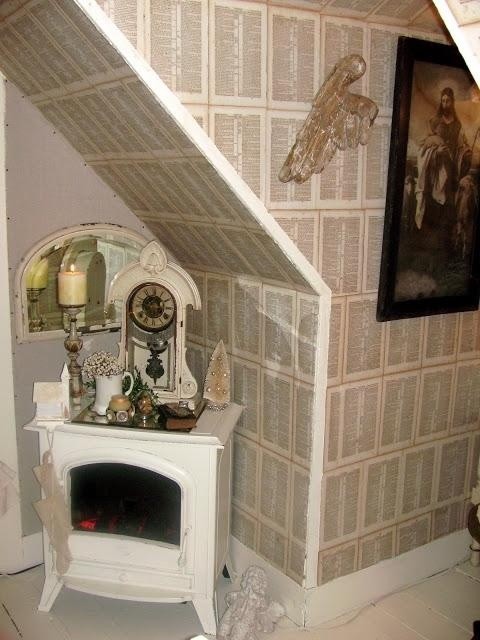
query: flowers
[{"left": 82, "top": 350, "right": 125, "bottom": 378}]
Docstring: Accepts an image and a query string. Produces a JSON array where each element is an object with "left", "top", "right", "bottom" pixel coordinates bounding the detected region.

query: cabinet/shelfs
[{"left": 22, "top": 403, "right": 245, "bottom": 637}]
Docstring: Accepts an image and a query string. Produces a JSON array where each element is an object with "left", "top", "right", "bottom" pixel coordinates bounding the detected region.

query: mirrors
[{"left": 15, "top": 222, "right": 151, "bottom": 345}]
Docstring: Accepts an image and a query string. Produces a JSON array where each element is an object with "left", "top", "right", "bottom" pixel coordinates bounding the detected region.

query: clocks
[{"left": 108, "top": 241, "right": 202, "bottom": 404}]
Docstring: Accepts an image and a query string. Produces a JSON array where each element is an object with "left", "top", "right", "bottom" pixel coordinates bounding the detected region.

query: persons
[{"left": 417, "top": 88, "right": 471, "bottom": 228}]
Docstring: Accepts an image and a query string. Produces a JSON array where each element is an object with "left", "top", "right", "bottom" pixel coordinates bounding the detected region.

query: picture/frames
[{"left": 376, "top": 36, "right": 480, "bottom": 322}]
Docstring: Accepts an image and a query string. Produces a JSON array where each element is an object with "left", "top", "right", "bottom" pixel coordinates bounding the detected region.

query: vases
[{"left": 90, "top": 371, "right": 134, "bottom": 416}]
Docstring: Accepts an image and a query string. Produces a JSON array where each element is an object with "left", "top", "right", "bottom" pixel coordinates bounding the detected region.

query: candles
[
  {"left": 26, "top": 258, "right": 48, "bottom": 288},
  {"left": 58, "top": 263, "right": 87, "bottom": 306}
]
